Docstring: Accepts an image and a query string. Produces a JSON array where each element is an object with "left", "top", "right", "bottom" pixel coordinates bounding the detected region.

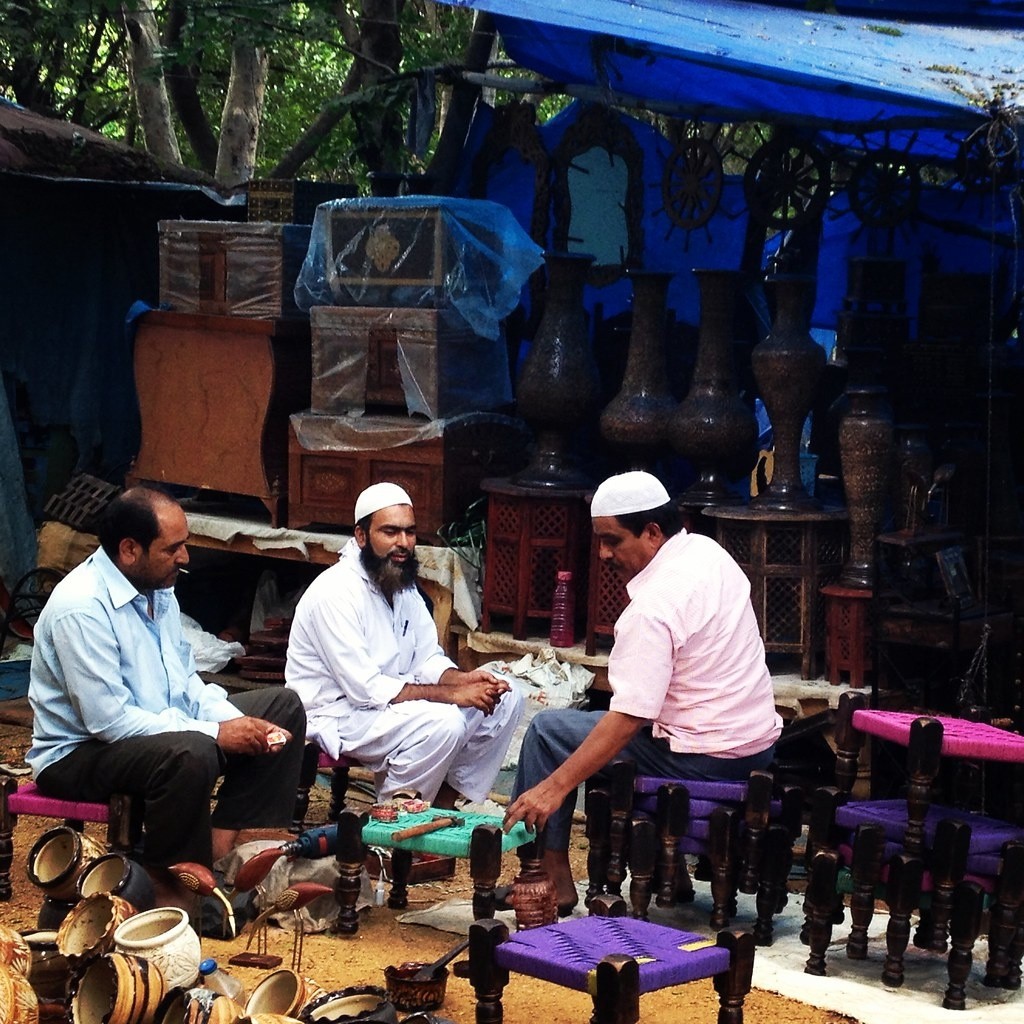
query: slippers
[
  {"left": 490, "top": 881, "right": 574, "bottom": 917},
  {"left": 648, "top": 877, "right": 696, "bottom": 906}
]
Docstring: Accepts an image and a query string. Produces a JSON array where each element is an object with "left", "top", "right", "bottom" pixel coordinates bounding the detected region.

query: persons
[
  {"left": 285, "top": 482, "right": 524, "bottom": 811},
  {"left": 24, "top": 486, "right": 307, "bottom": 933},
  {"left": 495, "top": 470, "right": 784, "bottom": 918}
]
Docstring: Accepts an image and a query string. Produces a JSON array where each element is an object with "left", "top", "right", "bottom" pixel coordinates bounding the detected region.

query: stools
[{"left": 7, "top": 685, "right": 1023, "bottom": 1024}]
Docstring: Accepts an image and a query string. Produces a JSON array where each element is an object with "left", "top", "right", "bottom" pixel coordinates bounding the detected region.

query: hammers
[{"left": 391, "top": 815, "right": 465, "bottom": 843}]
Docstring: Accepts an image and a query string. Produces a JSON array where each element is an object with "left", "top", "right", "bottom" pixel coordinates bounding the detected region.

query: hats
[
  {"left": 354, "top": 482, "right": 414, "bottom": 526},
  {"left": 590, "top": 470, "right": 671, "bottom": 519}
]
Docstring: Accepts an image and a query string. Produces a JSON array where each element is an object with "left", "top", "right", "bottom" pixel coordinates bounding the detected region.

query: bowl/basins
[{"left": 384, "top": 961, "right": 449, "bottom": 1013}]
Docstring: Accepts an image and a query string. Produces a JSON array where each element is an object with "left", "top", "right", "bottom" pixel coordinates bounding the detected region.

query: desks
[{"left": 172, "top": 517, "right": 482, "bottom": 675}]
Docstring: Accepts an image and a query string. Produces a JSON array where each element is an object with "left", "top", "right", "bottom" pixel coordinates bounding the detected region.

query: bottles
[
  {"left": 199, "top": 959, "right": 246, "bottom": 1007},
  {"left": 550, "top": 571, "right": 576, "bottom": 648}
]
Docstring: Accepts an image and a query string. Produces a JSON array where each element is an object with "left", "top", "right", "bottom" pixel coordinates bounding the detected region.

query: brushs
[{"left": 412, "top": 939, "right": 469, "bottom": 981}]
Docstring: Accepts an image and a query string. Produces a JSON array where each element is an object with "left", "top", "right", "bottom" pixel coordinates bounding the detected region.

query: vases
[{"left": 517, "top": 249, "right": 964, "bottom": 675}]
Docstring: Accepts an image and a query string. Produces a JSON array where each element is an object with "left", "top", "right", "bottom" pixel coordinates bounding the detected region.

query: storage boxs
[{"left": 158, "top": 198, "right": 511, "bottom": 534}]
